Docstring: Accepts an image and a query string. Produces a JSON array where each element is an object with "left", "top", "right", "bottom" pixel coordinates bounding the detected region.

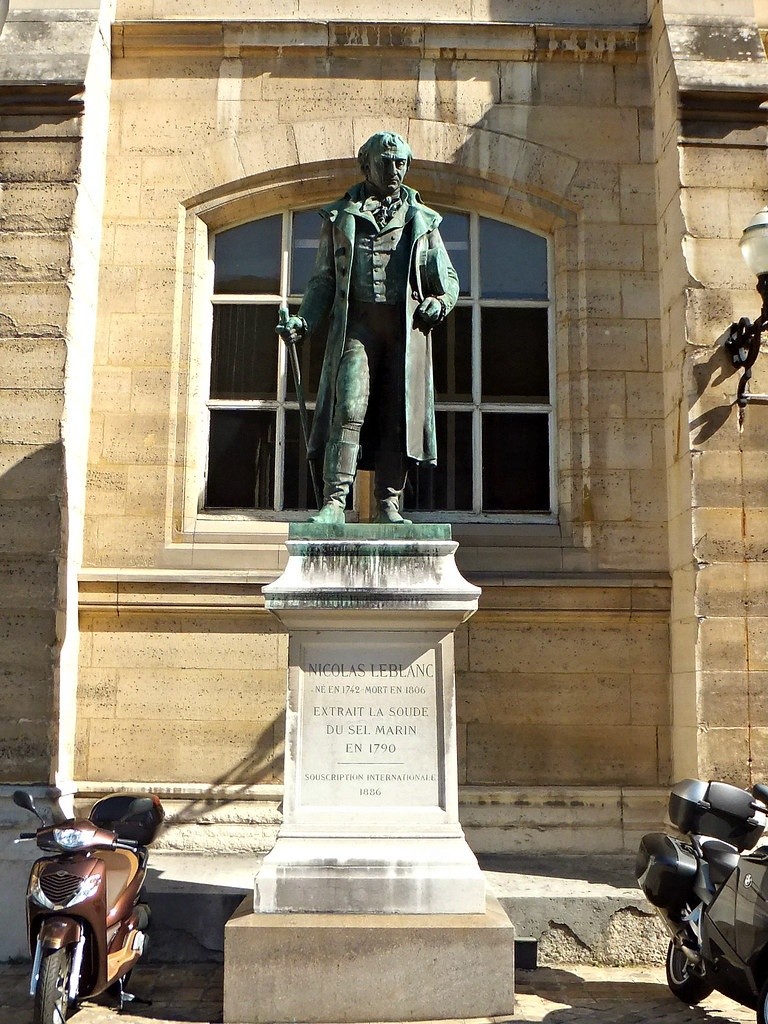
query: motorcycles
[{"left": 633, "top": 779, "right": 767, "bottom": 1024}]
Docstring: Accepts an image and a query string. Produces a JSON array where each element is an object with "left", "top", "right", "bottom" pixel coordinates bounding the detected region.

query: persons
[{"left": 274, "top": 131, "right": 459, "bottom": 524}]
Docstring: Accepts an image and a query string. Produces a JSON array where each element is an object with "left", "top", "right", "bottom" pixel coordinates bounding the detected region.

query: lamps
[{"left": 724, "top": 206, "right": 768, "bottom": 408}]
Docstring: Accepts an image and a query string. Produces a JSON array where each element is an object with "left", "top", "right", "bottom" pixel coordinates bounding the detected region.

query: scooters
[{"left": 8, "top": 789, "right": 167, "bottom": 1024}]
